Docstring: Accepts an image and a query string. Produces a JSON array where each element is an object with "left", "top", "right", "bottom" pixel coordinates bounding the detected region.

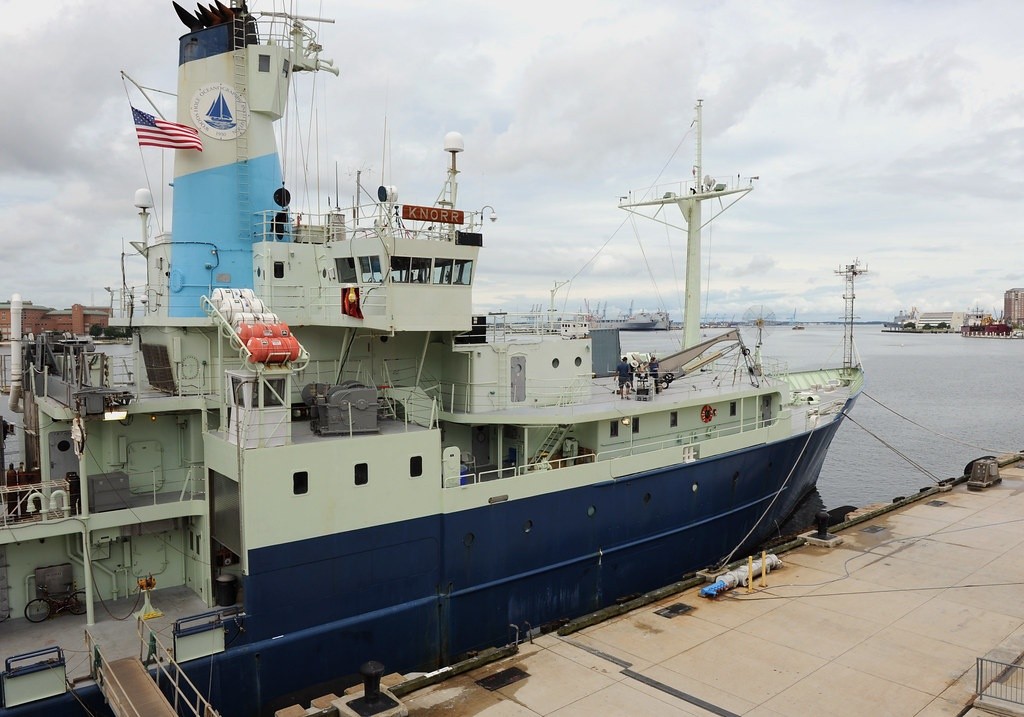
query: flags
[{"left": 131, "top": 106, "right": 203, "bottom": 151}]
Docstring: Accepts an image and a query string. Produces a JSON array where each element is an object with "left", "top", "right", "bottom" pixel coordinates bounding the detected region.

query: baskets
[{"left": 36, "top": 586, "right": 47, "bottom": 599}]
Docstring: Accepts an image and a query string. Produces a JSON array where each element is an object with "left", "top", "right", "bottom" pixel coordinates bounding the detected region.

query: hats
[{"left": 631, "top": 360, "right": 639, "bottom": 367}]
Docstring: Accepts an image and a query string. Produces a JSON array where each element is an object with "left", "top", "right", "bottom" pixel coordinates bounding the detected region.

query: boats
[{"left": 1, "top": 0, "right": 866, "bottom": 717}]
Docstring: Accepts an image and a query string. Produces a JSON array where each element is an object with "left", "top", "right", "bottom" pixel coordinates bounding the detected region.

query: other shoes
[{"left": 631, "top": 389, "right": 636, "bottom": 392}]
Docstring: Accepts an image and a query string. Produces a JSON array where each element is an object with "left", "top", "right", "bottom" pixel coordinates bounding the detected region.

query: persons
[{"left": 614, "top": 356, "right": 660, "bottom": 401}]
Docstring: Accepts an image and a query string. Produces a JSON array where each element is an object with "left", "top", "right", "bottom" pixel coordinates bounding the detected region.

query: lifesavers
[{"left": 701, "top": 405, "right": 713, "bottom": 423}]
